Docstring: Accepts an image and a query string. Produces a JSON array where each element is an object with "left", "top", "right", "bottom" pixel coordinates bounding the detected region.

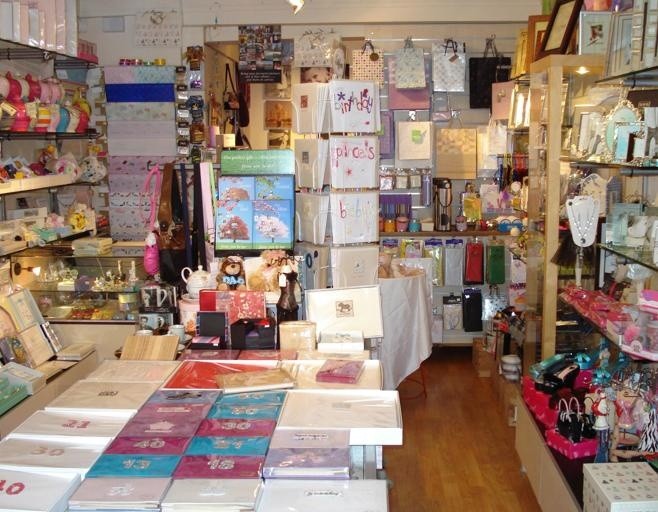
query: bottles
[
  {"left": 455, "top": 216, "right": 467, "bottom": 231},
  {"left": 434, "top": 177, "right": 452, "bottom": 231},
  {"left": 379, "top": 216, "right": 421, "bottom": 232},
  {"left": 501, "top": 354, "right": 523, "bottom": 382}
]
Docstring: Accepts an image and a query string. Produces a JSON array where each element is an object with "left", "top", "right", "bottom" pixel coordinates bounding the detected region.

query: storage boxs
[
  {"left": 582, "top": 461, "right": 658, "bottom": 512},
  {"left": 472, "top": 337, "right": 516, "bottom": 427}
]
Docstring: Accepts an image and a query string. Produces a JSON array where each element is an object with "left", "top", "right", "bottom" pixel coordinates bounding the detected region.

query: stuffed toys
[
  {"left": 215, "top": 256, "right": 246, "bottom": 291},
  {"left": 248, "top": 248, "right": 288, "bottom": 292}
]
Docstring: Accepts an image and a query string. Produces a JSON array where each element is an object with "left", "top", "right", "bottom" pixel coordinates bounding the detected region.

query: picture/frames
[
  {"left": 608, "top": 11, "right": 635, "bottom": 74},
  {"left": 516, "top": 29, "right": 531, "bottom": 75},
  {"left": 538, "top": 0, "right": 584, "bottom": 57},
  {"left": 579, "top": 11, "right": 612, "bottom": 55},
  {"left": 524, "top": 14, "right": 577, "bottom": 72}
]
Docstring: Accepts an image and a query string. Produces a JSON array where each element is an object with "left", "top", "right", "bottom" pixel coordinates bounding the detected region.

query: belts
[{"left": 156, "top": 159, "right": 211, "bottom": 279}]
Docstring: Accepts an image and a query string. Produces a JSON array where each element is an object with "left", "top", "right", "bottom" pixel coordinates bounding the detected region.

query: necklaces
[{"left": 568, "top": 198, "right": 596, "bottom": 244}]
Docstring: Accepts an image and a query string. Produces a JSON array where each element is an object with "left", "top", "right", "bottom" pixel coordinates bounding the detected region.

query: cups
[
  {"left": 141, "top": 286, "right": 167, "bottom": 309},
  {"left": 138, "top": 313, "right": 164, "bottom": 331},
  {"left": 167, "top": 323, "right": 185, "bottom": 340}
]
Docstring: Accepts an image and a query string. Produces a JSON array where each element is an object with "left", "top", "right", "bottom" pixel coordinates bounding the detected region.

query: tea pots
[{"left": 181, "top": 264, "right": 218, "bottom": 298}]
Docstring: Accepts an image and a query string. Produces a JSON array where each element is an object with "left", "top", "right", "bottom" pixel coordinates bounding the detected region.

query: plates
[
  {"left": 180, "top": 294, "right": 199, "bottom": 304},
  {"left": 161, "top": 334, "right": 193, "bottom": 352}
]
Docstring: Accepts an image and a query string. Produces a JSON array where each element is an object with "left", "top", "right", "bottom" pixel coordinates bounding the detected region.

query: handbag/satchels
[
  {"left": 350, "top": 27, "right": 518, "bottom": 181},
  {"left": 558, "top": 396, "right": 596, "bottom": 443}
]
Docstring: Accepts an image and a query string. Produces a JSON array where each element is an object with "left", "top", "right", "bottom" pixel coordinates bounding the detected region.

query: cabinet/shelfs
[
  {"left": 10, "top": 241, "right": 147, "bottom": 322},
  {"left": 0, "top": 38, "right": 109, "bottom": 259},
  {"left": 511, "top": 55, "right": 658, "bottom": 512}
]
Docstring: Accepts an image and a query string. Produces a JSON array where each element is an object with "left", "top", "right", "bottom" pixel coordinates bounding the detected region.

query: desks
[{"left": 379, "top": 267, "right": 436, "bottom": 391}]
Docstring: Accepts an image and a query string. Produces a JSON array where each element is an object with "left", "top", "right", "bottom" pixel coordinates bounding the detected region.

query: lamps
[{"left": 14, "top": 263, "right": 40, "bottom": 276}]
